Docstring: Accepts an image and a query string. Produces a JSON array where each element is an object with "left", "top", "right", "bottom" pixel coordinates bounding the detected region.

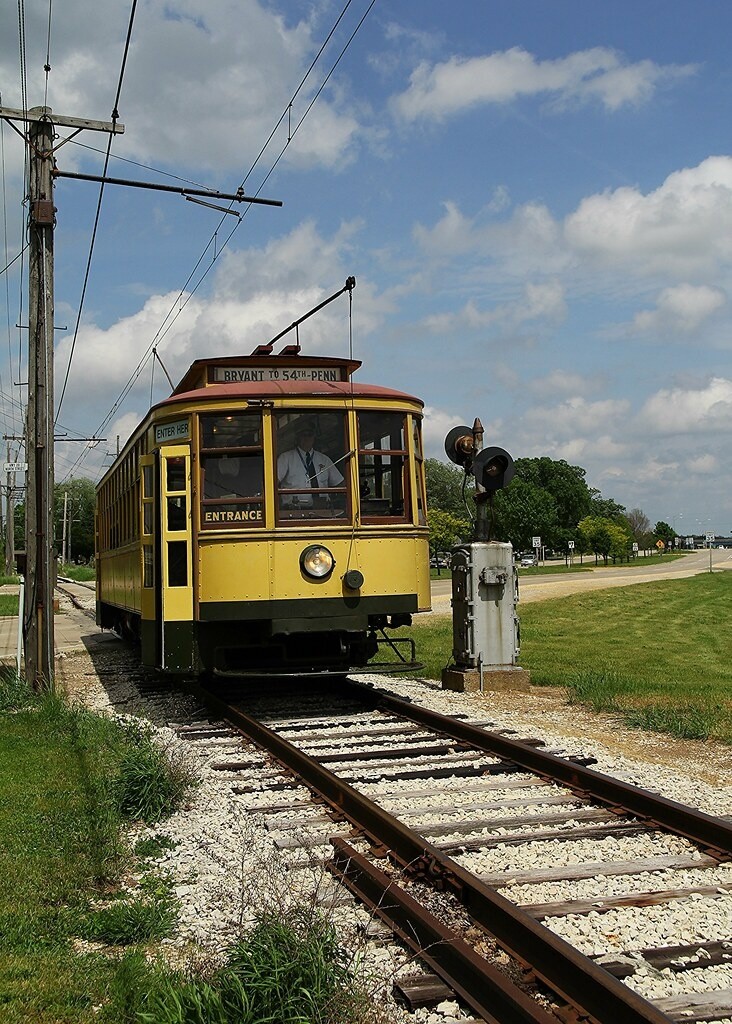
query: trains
[{"left": 96, "top": 276, "right": 433, "bottom": 689}]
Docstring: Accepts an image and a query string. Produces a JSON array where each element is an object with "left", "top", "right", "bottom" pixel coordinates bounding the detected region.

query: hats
[{"left": 295, "top": 421, "right": 317, "bottom": 434}]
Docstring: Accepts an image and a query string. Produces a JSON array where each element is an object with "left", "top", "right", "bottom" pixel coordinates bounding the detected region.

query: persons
[{"left": 274, "top": 420, "right": 348, "bottom": 511}]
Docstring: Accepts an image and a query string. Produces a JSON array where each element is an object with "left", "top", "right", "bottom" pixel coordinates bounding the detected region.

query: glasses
[{"left": 300, "top": 432, "right": 315, "bottom": 437}]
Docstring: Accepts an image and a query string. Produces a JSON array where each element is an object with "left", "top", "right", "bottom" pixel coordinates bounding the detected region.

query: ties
[{"left": 306, "top": 452, "right": 319, "bottom": 500}]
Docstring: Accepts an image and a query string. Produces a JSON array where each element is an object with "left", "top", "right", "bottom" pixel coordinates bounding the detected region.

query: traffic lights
[{"left": 473, "top": 446, "right": 515, "bottom": 491}]
